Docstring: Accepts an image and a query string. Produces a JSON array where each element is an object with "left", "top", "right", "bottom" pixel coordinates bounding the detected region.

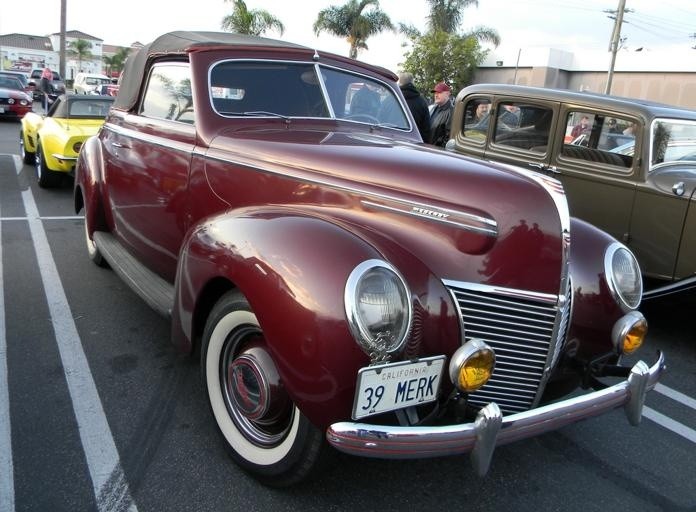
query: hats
[{"left": 431, "top": 83, "right": 450, "bottom": 93}]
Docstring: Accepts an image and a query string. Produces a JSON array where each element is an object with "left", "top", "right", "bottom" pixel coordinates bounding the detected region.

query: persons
[
  {"left": 623, "top": 123, "right": 637, "bottom": 140},
  {"left": 38, "top": 68, "right": 54, "bottom": 115},
  {"left": 427, "top": 83, "right": 455, "bottom": 149},
  {"left": 606, "top": 119, "right": 624, "bottom": 143},
  {"left": 464, "top": 101, "right": 522, "bottom": 129},
  {"left": 375, "top": 72, "right": 431, "bottom": 147},
  {"left": 348, "top": 84, "right": 382, "bottom": 124},
  {"left": 570, "top": 116, "right": 591, "bottom": 139}
]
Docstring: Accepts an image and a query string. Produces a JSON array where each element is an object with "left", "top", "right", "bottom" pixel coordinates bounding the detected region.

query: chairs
[{"left": 494, "top": 132, "right": 627, "bottom": 167}]
